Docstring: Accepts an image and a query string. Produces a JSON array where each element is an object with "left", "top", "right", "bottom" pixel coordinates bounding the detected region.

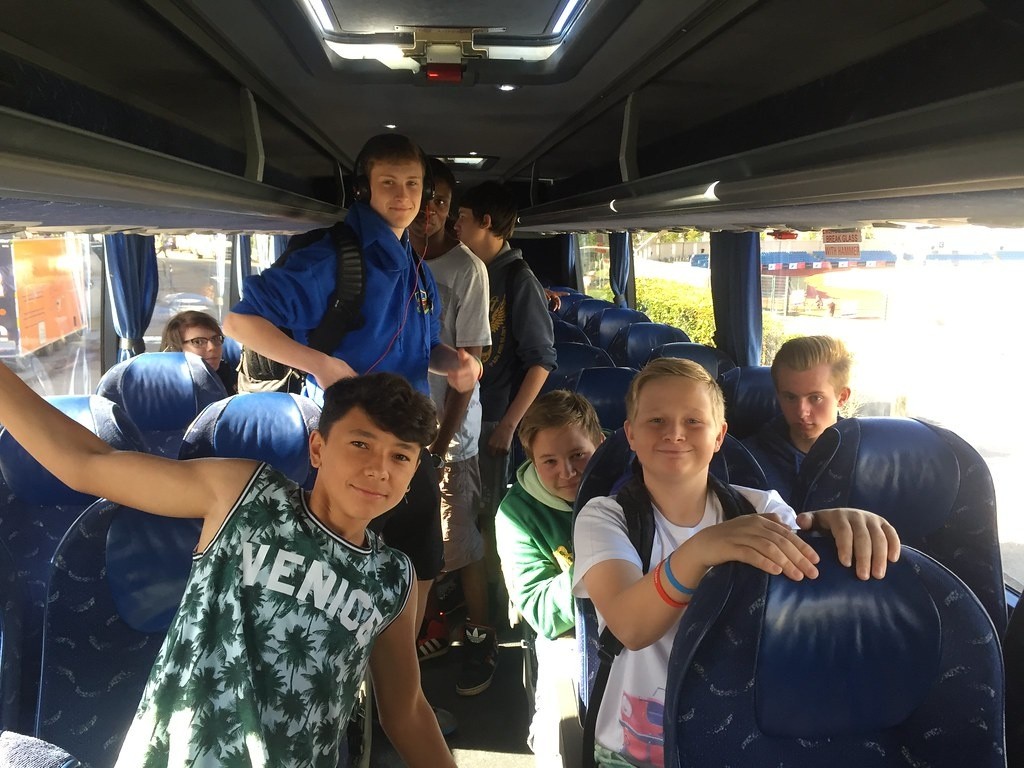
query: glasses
[{"left": 182, "top": 335, "right": 225, "bottom": 348}]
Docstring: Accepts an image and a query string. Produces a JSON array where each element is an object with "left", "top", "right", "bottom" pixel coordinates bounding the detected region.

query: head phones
[{"left": 351, "top": 133, "right": 436, "bottom": 208}]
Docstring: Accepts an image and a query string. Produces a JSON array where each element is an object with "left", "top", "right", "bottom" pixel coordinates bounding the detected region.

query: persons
[
  {"left": 157, "top": 309, "right": 235, "bottom": 400},
  {"left": 494, "top": 389, "right": 608, "bottom": 768},
  {"left": 542, "top": 286, "right": 572, "bottom": 312},
  {"left": 2, "top": 358, "right": 458, "bottom": 768},
  {"left": 445, "top": 182, "right": 558, "bottom": 635},
  {"left": 219, "top": 132, "right": 485, "bottom": 737},
  {"left": 732, "top": 335, "right": 853, "bottom": 514},
  {"left": 569, "top": 355, "right": 902, "bottom": 768},
  {"left": 406, "top": 157, "right": 499, "bottom": 696}
]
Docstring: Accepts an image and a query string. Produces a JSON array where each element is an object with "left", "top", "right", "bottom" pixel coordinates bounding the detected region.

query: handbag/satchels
[{"left": 236, "top": 371, "right": 290, "bottom": 395}]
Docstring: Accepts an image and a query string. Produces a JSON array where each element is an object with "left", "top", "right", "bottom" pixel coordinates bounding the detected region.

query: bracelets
[
  {"left": 654, "top": 556, "right": 689, "bottom": 609},
  {"left": 664, "top": 551, "right": 695, "bottom": 595}
]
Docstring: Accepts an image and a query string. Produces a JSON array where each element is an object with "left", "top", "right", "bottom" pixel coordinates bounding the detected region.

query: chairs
[{"left": 0, "top": 282, "right": 1004, "bottom": 768}]
